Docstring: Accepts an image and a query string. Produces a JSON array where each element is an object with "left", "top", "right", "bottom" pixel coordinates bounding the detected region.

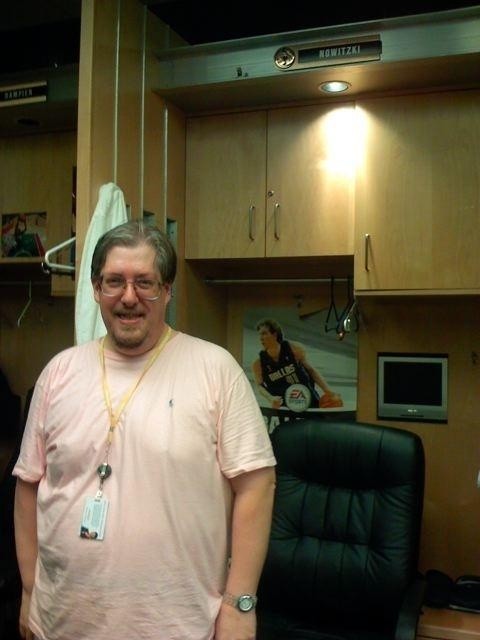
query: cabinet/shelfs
[
  {"left": 180, "top": 97, "right": 358, "bottom": 272},
  {"left": 354, "top": 85, "right": 480, "bottom": 304}
]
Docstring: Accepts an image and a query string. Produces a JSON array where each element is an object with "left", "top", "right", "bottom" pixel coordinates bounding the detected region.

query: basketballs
[{"left": 319, "top": 392, "right": 343, "bottom": 408}]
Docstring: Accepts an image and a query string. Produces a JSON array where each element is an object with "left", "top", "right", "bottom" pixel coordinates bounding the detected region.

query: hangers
[
  {"left": 40, "top": 234, "right": 74, "bottom": 278},
  {"left": 321, "top": 274, "right": 360, "bottom": 339}
]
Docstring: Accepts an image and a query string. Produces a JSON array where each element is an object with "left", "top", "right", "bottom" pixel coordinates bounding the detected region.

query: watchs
[{"left": 220, "top": 590, "right": 259, "bottom": 613}]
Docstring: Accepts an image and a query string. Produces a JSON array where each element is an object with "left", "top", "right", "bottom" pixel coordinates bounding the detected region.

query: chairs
[{"left": 256, "top": 416, "right": 428, "bottom": 638}]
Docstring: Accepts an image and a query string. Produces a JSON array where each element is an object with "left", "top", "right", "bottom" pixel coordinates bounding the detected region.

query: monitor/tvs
[{"left": 377, "top": 354, "right": 448, "bottom": 422}]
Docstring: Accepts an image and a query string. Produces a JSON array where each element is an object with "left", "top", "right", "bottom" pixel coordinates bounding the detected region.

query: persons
[
  {"left": 11, "top": 219, "right": 277, "bottom": 640},
  {"left": 249, "top": 318, "right": 343, "bottom": 408},
  {"left": 80, "top": 526, "right": 98, "bottom": 539}
]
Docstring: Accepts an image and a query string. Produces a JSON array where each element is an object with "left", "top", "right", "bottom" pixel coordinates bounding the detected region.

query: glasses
[{"left": 98, "top": 281, "right": 167, "bottom": 302}]
